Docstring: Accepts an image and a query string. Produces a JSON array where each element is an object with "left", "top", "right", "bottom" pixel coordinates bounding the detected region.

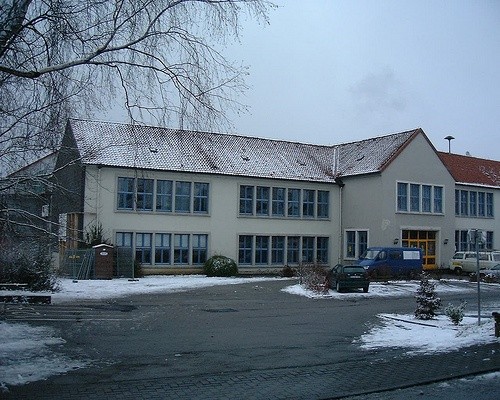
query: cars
[{"left": 324, "top": 263, "right": 369, "bottom": 294}]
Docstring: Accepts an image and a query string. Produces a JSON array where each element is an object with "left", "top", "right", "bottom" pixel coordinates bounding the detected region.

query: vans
[
  {"left": 356, "top": 246, "right": 423, "bottom": 279},
  {"left": 451, "top": 250, "right": 500, "bottom": 277}
]
